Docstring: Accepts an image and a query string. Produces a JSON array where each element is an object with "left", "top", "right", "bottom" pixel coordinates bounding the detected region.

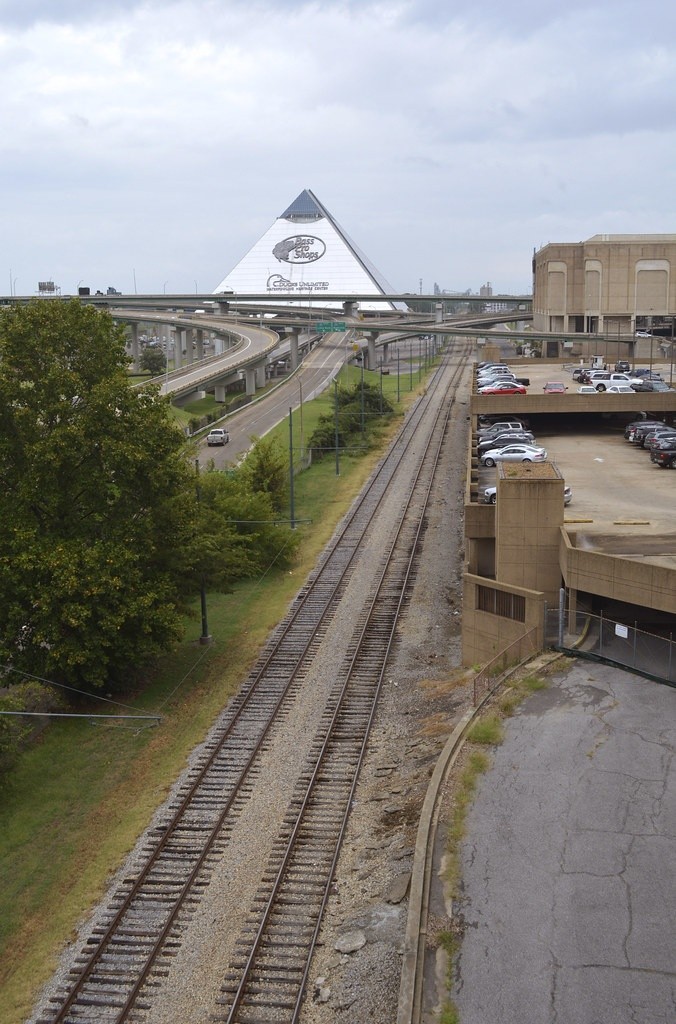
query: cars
[
  {"left": 624, "top": 369, "right": 660, "bottom": 377},
  {"left": 576, "top": 385, "right": 599, "bottom": 394},
  {"left": 638, "top": 374, "right": 665, "bottom": 381},
  {"left": 419, "top": 336, "right": 433, "bottom": 340},
  {"left": 139, "top": 335, "right": 210, "bottom": 351},
  {"left": 481, "top": 383, "right": 527, "bottom": 396},
  {"left": 484, "top": 484, "right": 571, "bottom": 507},
  {"left": 606, "top": 386, "right": 637, "bottom": 393},
  {"left": 476, "top": 361, "right": 526, "bottom": 394},
  {"left": 480, "top": 443, "right": 548, "bottom": 467}
]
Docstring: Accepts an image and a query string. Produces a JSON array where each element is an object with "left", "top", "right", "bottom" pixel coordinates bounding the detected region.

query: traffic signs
[{"left": 316, "top": 321, "right": 345, "bottom": 333}]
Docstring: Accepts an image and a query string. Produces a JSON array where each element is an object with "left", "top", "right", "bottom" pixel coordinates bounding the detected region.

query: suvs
[
  {"left": 580, "top": 369, "right": 608, "bottom": 384},
  {"left": 476, "top": 436, "right": 532, "bottom": 457},
  {"left": 476, "top": 413, "right": 536, "bottom": 445},
  {"left": 572, "top": 369, "right": 590, "bottom": 382},
  {"left": 615, "top": 360, "right": 630, "bottom": 372},
  {"left": 543, "top": 381, "right": 568, "bottom": 395},
  {"left": 590, "top": 373, "right": 626, "bottom": 380},
  {"left": 624, "top": 422, "right": 676, "bottom": 450}
]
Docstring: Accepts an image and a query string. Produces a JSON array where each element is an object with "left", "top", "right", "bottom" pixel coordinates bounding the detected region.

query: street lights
[
  {"left": 593, "top": 318, "right": 624, "bottom": 366},
  {"left": 164, "top": 281, "right": 168, "bottom": 294},
  {"left": 588, "top": 294, "right": 592, "bottom": 357},
  {"left": 322, "top": 303, "right": 330, "bottom": 337},
  {"left": 369, "top": 305, "right": 380, "bottom": 323},
  {"left": 192, "top": 280, "right": 197, "bottom": 296},
  {"left": 629, "top": 320, "right": 636, "bottom": 371},
  {"left": 666, "top": 316, "right": 675, "bottom": 387},
  {"left": 10, "top": 268, "right": 16, "bottom": 297},
  {"left": 353, "top": 341, "right": 364, "bottom": 383},
  {"left": 133, "top": 268, "right": 137, "bottom": 294},
  {"left": 225, "top": 286, "right": 237, "bottom": 325},
  {"left": 645, "top": 316, "right": 653, "bottom": 380},
  {"left": 77, "top": 280, "right": 83, "bottom": 295},
  {"left": 166, "top": 317, "right": 175, "bottom": 396}
]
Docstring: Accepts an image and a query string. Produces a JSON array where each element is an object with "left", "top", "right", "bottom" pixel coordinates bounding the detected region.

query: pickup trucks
[
  {"left": 478, "top": 376, "right": 530, "bottom": 388},
  {"left": 632, "top": 380, "right": 675, "bottom": 392},
  {"left": 589, "top": 374, "right": 645, "bottom": 392},
  {"left": 651, "top": 449, "right": 676, "bottom": 469},
  {"left": 207, "top": 429, "right": 230, "bottom": 446}
]
